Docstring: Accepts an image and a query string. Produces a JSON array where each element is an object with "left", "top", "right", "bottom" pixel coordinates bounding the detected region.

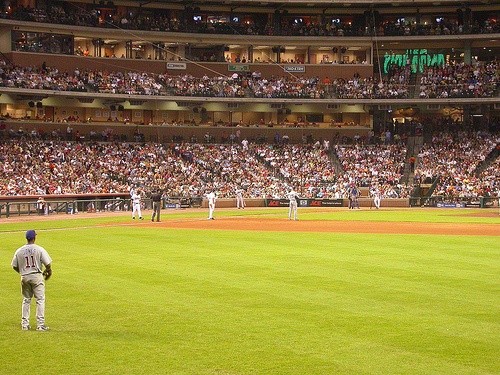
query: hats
[{"left": 26, "top": 230, "right": 37, "bottom": 239}]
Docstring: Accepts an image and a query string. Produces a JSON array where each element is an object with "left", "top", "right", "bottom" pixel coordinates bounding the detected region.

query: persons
[
  {"left": 0, "top": 4, "right": 500, "bottom": 36},
  {"left": 289, "top": 187, "right": 299, "bottom": 221},
  {"left": 131, "top": 191, "right": 144, "bottom": 221},
  {"left": 208, "top": 190, "right": 217, "bottom": 220},
  {"left": 11, "top": 230, "right": 52, "bottom": 331},
  {"left": 150, "top": 189, "right": 162, "bottom": 222},
  {"left": 0, "top": 54, "right": 500, "bottom": 99},
  {"left": 0, "top": 114, "right": 500, "bottom": 215}
]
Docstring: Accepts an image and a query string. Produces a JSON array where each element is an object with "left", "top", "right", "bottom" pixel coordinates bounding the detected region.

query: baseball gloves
[{"left": 43, "top": 268, "right": 52, "bottom": 280}]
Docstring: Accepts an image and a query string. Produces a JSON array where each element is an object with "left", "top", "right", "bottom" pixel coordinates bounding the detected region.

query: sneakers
[
  {"left": 22, "top": 325, "right": 31, "bottom": 330},
  {"left": 36, "top": 326, "right": 49, "bottom": 330}
]
